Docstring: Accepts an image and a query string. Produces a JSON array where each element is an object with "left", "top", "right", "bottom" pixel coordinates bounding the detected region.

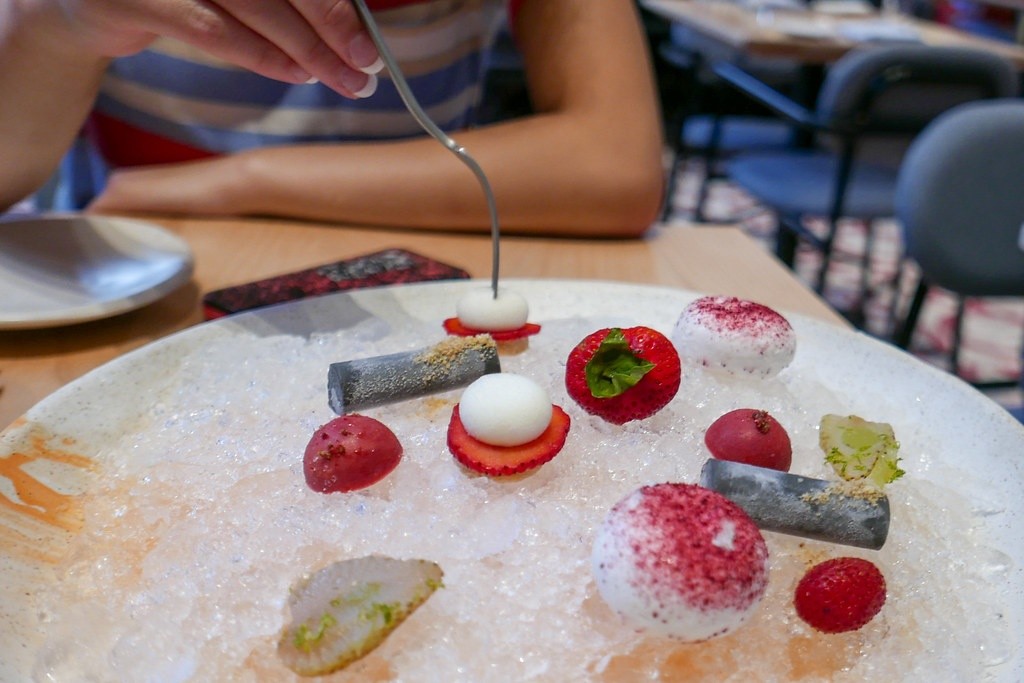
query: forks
[{"left": 347, "top": 0, "right": 502, "bottom": 304}]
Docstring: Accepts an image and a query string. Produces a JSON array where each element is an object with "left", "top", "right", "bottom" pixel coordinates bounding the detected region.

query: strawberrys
[
  {"left": 447, "top": 402, "right": 571, "bottom": 477},
  {"left": 564, "top": 326, "right": 682, "bottom": 425},
  {"left": 443, "top": 318, "right": 541, "bottom": 341}
]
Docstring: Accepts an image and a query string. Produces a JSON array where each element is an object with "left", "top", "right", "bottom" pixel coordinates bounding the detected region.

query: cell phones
[{"left": 198, "top": 247, "right": 471, "bottom": 322}]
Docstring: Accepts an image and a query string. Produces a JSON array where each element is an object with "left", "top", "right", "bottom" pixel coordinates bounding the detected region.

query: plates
[
  {"left": 0, "top": 279, "right": 1024, "bottom": 683},
  {"left": 0, "top": 212, "right": 195, "bottom": 333}
]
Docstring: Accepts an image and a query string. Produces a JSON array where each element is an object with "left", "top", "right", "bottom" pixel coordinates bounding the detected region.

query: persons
[{"left": 1, "top": 0, "right": 664, "bottom": 241}]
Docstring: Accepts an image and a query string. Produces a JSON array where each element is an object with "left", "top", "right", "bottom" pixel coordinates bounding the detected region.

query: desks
[
  {"left": 642, "top": 0, "right": 1024, "bottom": 277},
  {"left": 0, "top": 216, "right": 851, "bottom": 429}
]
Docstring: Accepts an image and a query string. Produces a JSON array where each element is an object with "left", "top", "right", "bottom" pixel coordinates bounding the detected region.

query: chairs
[{"left": 664, "top": 46, "right": 1024, "bottom": 387}]
